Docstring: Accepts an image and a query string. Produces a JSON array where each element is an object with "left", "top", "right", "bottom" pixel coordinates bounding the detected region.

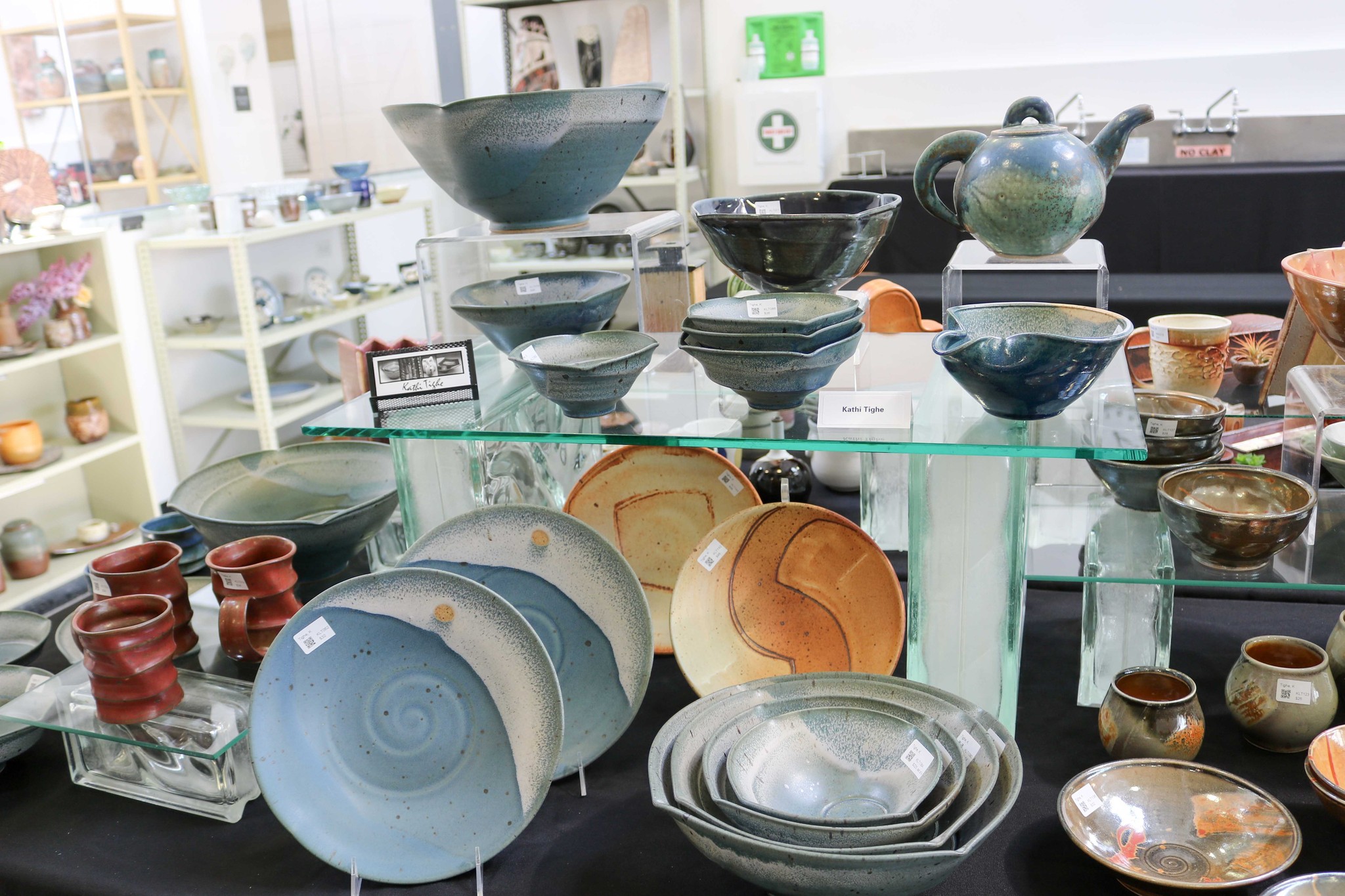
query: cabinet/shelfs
[{"left": 0, "top": 1, "right": 719, "bottom": 629}]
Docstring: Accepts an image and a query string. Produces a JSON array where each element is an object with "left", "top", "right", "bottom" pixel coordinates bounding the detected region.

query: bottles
[
  {"left": 1224, "top": 636, "right": 1338, "bottom": 754},
  {"left": 40, "top": 48, "right": 168, "bottom": 96},
  {"left": 1098, "top": 668, "right": 1205, "bottom": 760},
  {"left": 748, "top": 417, "right": 812, "bottom": 503},
  {"left": 1326, "top": 610, "right": 1345, "bottom": 706}
]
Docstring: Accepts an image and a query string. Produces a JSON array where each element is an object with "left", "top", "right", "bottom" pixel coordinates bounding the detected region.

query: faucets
[
  {"left": 1053, "top": 91, "right": 1096, "bottom": 142},
  {"left": 1165, "top": 84, "right": 1251, "bottom": 137}
]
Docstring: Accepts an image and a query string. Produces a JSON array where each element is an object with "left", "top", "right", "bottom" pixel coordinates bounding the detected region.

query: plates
[
  {"left": 307, "top": 330, "right": 348, "bottom": 380},
  {"left": 49, "top": 523, "right": 139, "bottom": 558},
  {"left": 247, "top": 439, "right": 904, "bottom": 886},
  {"left": 237, "top": 382, "right": 318, "bottom": 406},
  {"left": 1258, "top": 872, "right": 1345, "bottom": 896},
  {"left": 1059, "top": 759, "right": 1302, "bottom": 888}
]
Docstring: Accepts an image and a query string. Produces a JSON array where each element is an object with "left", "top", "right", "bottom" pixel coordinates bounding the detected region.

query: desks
[
  {"left": 799, "top": 115, "right": 1345, "bottom": 323},
  {"left": 0, "top": 341, "right": 1345, "bottom": 896}
]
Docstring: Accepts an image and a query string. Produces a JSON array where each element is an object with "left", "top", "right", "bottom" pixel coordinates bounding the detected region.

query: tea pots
[{"left": 912, "top": 94, "right": 1154, "bottom": 258}]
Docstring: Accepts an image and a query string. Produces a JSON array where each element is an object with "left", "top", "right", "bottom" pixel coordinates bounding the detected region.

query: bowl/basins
[
  {"left": 650, "top": 674, "right": 1023, "bottom": 896},
  {"left": 199, "top": 162, "right": 422, "bottom": 303},
  {"left": 931, "top": 304, "right": 1134, "bottom": 420},
  {"left": 690, "top": 191, "right": 901, "bottom": 295},
  {"left": 1281, "top": 246, "right": 1345, "bottom": 365},
  {"left": 382, "top": 90, "right": 664, "bottom": 420},
  {"left": 1305, "top": 725, "right": 1345, "bottom": 822},
  {"left": 163, "top": 440, "right": 398, "bottom": 579},
  {"left": 1080, "top": 389, "right": 1345, "bottom": 571},
  {"left": 0, "top": 611, "right": 56, "bottom": 762},
  {"left": 676, "top": 292, "right": 866, "bottom": 412}
]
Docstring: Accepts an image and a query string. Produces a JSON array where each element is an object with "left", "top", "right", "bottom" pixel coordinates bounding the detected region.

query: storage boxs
[{"left": 729, "top": 74, "right": 852, "bottom": 195}]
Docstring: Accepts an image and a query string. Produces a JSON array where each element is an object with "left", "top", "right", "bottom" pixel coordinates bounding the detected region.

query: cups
[
  {"left": 1122, "top": 312, "right": 1234, "bottom": 398},
  {"left": 0, "top": 396, "right": 109, "bottom": 597},
  {"left": 804, "top": 417, "right": 861, "bottom": 492},
  {"left": 68, "top": 540, "right": 303, "bottom": 723}
]
